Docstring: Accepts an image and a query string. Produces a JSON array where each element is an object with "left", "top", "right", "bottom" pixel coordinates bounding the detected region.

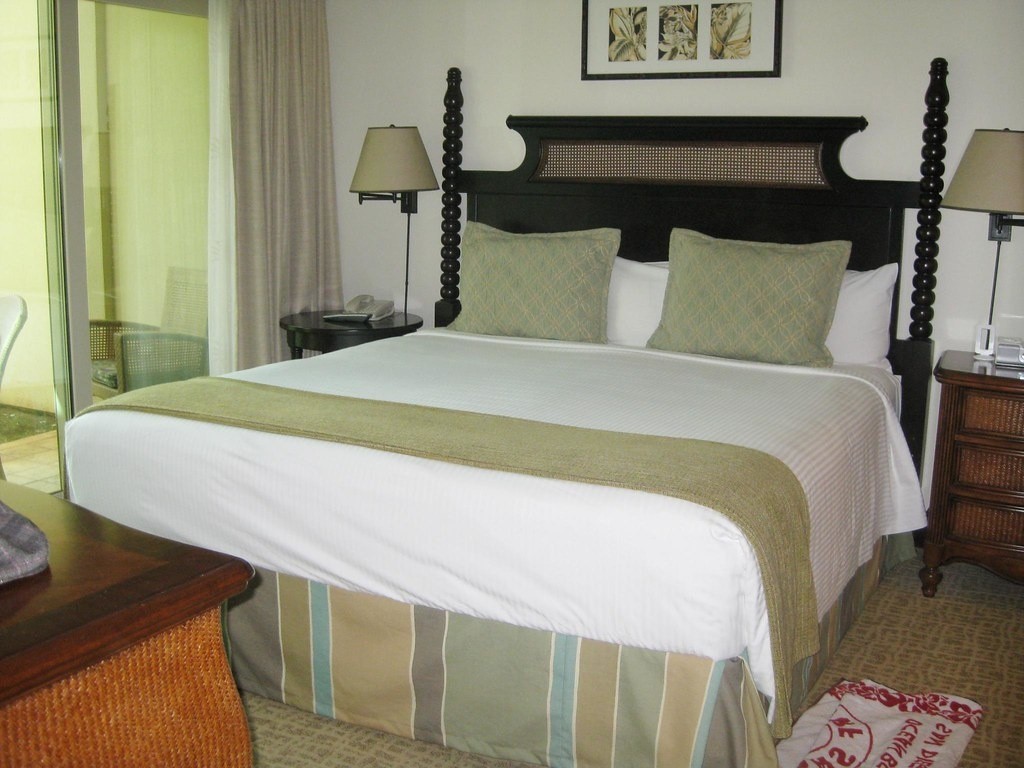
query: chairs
[
  {"left": 88, "top": 266, "right": 208, "bottom": 400},
  {"left": 0, "top": 294, "right": 28, "bottom": 482}
]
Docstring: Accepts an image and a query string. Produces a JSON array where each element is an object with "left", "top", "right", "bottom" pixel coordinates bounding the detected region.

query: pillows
[
  {"left": 606, "top": 255, "right": 669, "bottom": 349},
  {"left": 442, "top": 220, "right": 621, "bottom": 344},
  {"left": 645, "top": 227, "right": 852, "bottom": 368},
  {"left": 825, "top": 263, "right": 898, "bottom": 375}
]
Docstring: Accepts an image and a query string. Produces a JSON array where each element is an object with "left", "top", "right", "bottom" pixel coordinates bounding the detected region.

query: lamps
[
  {"left": 348, "top": 124, "right": 440, "bottom": 314},
  {"left": 941, "top": 128, "right": 1024, "bottom": 350}
]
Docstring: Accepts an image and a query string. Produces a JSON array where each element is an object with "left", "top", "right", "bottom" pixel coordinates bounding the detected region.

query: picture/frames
[{"left": 580, "top": 0, "right": 784, "bottom": 81}]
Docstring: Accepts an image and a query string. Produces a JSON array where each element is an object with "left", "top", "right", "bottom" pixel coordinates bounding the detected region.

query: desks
[{"left": 0, "top": 481, "right": 256, "bottom": 768}]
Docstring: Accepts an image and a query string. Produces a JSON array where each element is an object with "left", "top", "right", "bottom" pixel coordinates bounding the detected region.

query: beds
[{"left": 64, "top": 57, "right": 951, "bottom": 768}]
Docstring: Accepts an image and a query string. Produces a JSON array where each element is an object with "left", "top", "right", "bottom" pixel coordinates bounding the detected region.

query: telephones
[{"left": 343, "top": 295, "right": 395, "bottom": 321}]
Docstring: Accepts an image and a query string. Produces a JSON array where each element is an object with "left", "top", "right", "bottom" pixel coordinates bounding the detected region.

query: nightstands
[
  {"left": 279, "top": 309, "right": 423, "bottom": 361},
  {"left": 918, "top": 350, "right": 1023, "bottom": 598}
]
